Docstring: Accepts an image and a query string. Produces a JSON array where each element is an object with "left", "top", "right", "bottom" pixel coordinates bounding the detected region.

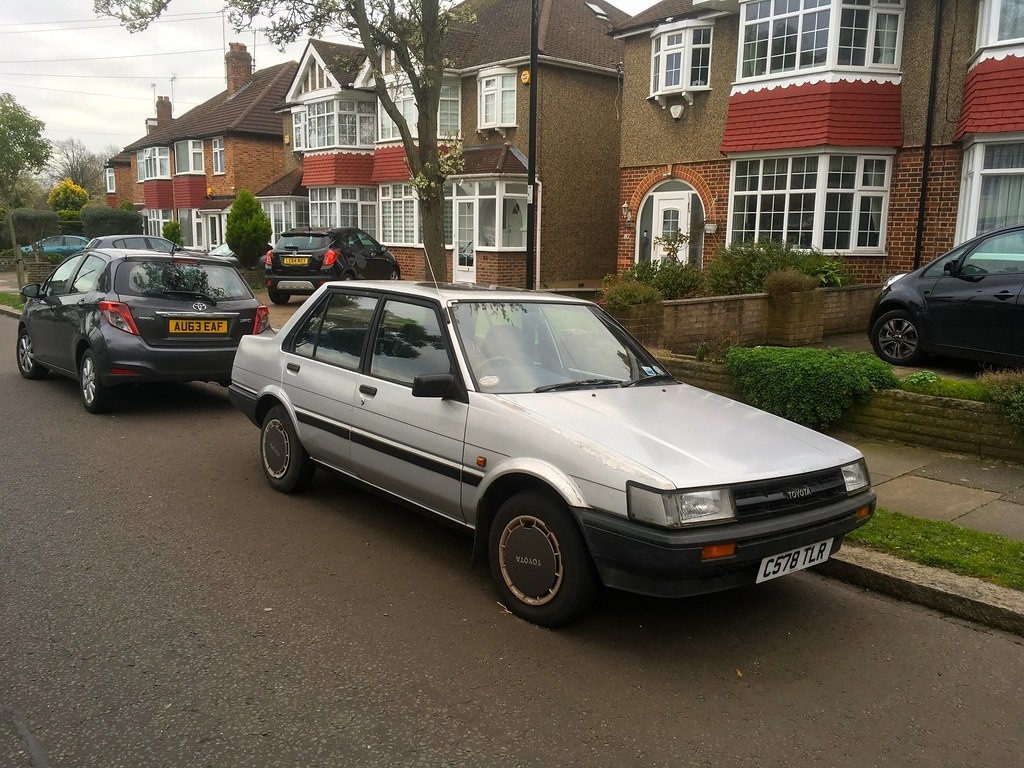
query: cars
[
  {"left": 227, "top": 276, "right": 878, "bottom": 630},
  {"left": 19, "top": 234, "right": 89, "bottom": 258},
  {"left": 866, "top": 225, "right": 1024, "bottom": 372},
  {"left": 81, "top": 234, "right": 189, "bottom": 251},
  {"left": 13, "top": 246, "right": 272, "bottom": 416},
  {"left": 208, "top": 240, "right": 274, "bottom": 269}
]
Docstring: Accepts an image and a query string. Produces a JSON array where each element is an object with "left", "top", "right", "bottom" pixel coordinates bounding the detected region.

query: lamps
[
  {"left": 662, "top": 172, "right": 673, "bottom": 179},
  {"left": 615, "top": 61, "right": 623, "bottom": 73},
  {"left": 621, "top": 201, "right": 631, "bottom": 222}
]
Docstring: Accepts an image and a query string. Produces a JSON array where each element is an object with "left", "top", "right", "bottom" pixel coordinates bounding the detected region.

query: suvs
[{"left": 263, "top": 225, "right": 403, "bottom": 306}]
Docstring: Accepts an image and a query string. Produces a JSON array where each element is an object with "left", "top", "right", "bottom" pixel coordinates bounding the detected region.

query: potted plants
[{"left": 686, "top": 220, "right": 721, "bottom": 245}]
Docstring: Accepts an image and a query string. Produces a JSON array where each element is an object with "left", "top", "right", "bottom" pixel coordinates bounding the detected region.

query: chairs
[
  {"left": 478, "top": 324, "right": 524, "bottom": 367},
  {"left": 393, "top": 323, "right": 441, "bottom": 369}
]
